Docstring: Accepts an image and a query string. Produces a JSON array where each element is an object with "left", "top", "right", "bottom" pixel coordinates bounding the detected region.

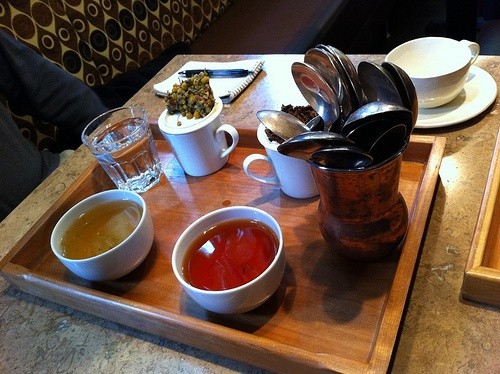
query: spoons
[{"left": 256, "top": 43, "right": 419, "bottom": 170}]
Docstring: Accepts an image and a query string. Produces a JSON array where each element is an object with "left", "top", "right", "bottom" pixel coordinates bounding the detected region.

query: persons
[{"left": 0, "top": 30, "right": 112, "bottom": 222}]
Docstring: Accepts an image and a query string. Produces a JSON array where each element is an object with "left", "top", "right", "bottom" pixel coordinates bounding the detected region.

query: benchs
[{"left": 0, "top": 0, "right": 353, "bottom": 154}]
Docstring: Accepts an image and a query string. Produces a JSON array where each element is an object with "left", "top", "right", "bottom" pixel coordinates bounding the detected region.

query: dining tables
[{"left": 0, "top": 54, "right": 500, "bottom": 374}]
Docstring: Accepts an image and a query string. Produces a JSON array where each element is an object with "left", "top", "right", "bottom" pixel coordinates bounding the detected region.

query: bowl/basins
[
  {"left": 49, "top": 189, "right": 155, "bottom": 282},
  {"left": 171, "top": 205, "right": 287, "bottom": 317}
]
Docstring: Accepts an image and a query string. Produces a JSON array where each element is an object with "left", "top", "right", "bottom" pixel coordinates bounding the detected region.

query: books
[{"left": 153, "top": 59, "right": 265, "bottom": 103}]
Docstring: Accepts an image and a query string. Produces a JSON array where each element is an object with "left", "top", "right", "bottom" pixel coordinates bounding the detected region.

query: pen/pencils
[{"left": 177, "top": 69, "right": 255, "bottom": 78}]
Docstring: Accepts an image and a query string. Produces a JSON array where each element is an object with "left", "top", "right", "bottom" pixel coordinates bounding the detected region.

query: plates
[{"left": 414, "top": 65, "right": 497, "bottom": 128}]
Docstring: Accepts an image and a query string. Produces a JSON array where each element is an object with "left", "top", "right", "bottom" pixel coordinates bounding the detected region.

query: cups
[
  {"left": 157, "top": 96, "right": 240, "bottom": 178},
  {"left": 384, "top": 36, "right": 481, "bottom": 109},
  {"left": 242, "top": 122, "right": 320, "bottom": 200},
  {"left": 309, "top": 152, "right": 409, "bottom": 262},
  {"left": 81, "top": 106, "right": 162, "bottom": 194}
]
